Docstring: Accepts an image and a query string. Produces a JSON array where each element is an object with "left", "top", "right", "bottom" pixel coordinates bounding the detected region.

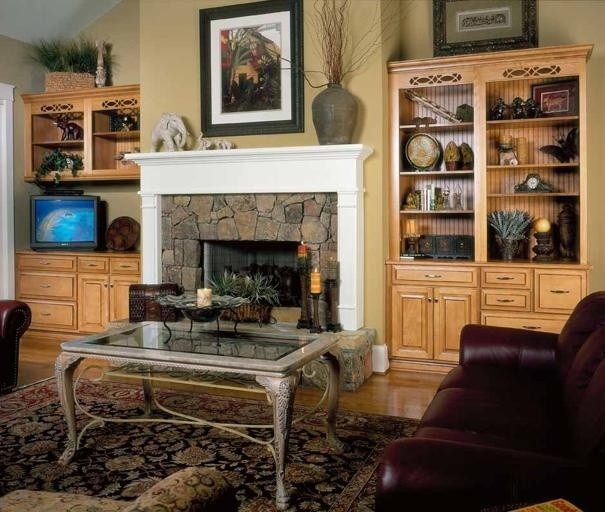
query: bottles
[
  {"left": 442, "top": 184, "right": 451, "bottom": 210},
  {"left": 429, "top": 196, "right": 437, "bottom": 210},
  {"left": 421, "top": 184, "right": 432, "bottom": 211}
]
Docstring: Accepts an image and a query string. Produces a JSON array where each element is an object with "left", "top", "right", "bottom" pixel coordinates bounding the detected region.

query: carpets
[{"left": 1, "top": 374, "right": 421, "bottom": 511}]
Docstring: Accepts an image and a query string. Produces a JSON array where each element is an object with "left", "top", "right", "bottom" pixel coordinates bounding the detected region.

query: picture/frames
[
  {"left": 433, "top": 0, "right": 539, "bottom": 55},
  {"left": 198, "top": 0, "right": 303, "bottom": 137}
]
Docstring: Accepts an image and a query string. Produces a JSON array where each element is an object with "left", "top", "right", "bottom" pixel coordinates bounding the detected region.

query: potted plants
[
  {"left": 213, "top": 272, "right": 279, "bottom": 320},
  {"left": 21, "top": 33, "right": 119, "bottom": 92},
  {"left": 491, "top": 209, "right": 531, "bottom": 260},
  {"left": 263, "top": 1, "right": 416, "bottom": 145}
]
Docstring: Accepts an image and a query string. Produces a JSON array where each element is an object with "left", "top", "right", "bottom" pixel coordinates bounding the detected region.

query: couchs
[
  {"left": 0, "top": 297, "right": 33, "bottom": 395},
  {"left": 377, "top": 290, "right": 605, "bottom": 510}
]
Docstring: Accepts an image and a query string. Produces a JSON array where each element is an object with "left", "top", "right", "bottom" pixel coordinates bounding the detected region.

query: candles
[
  {"left": 198, "top": 288, "right": 213, "bottom": 309},
  {"left": 310, "top": 268, "right": 322, "bottom": 294},
  {"left": 299, "top": 243, "right": 309, "bottom": 260}
]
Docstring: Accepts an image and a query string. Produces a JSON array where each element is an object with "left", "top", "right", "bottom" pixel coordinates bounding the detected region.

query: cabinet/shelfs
[
  {"left": 17, "top": 89, "right": 143, "bottom": 332},
  {"left": 386, "top": 43, "right": 594, "bottom": 375}
]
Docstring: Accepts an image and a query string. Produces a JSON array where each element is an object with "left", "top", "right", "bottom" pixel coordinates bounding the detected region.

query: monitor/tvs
[{"left": 28, "top": 194, "right": 99, "bottom": 254}]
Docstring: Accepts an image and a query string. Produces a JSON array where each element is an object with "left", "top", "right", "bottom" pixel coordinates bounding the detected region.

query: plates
[
  {"left": 404, "top": 133, "right": 442, "bottom": 170},
  {"left": 105, "top": 216, "right": 140, "bottom": 252}
]
